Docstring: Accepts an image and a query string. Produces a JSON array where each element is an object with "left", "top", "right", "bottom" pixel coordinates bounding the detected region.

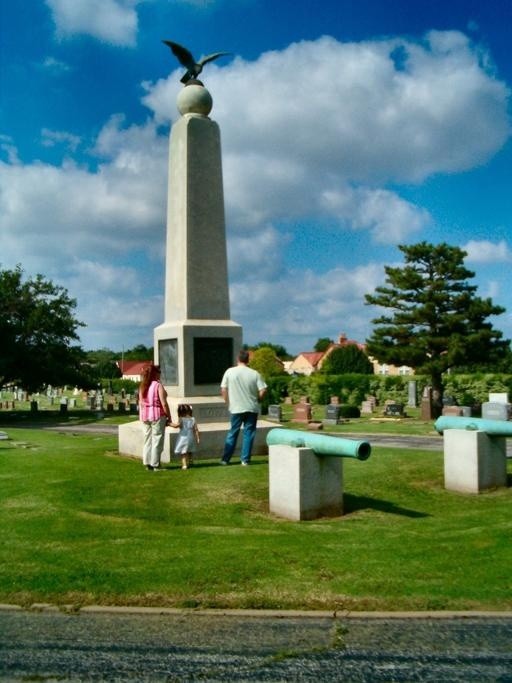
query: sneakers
[
  {"left": 145, "top": 464, "right": 167, "bottom": 471},
  {"left": 241, "top": 460, "right": 250, "bottom": 466},
  {"left": 220, "top": 460, "right": 230, "bottom": 465}
]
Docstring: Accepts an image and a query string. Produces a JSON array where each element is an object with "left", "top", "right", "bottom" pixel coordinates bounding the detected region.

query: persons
[
  {"left": 220, "top": 351, "right": 268, "bottom": 466},
  {"left": 139, "top": 365, "right": 172, "bottom": 472},
  {"left": 166, "top": 404, "right": 200, "bottom": 470}
]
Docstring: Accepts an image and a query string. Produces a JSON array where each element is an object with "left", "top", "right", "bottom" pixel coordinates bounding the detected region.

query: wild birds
[{"left": 161, "top": 40, "right": 233, "bottom": 84}]
